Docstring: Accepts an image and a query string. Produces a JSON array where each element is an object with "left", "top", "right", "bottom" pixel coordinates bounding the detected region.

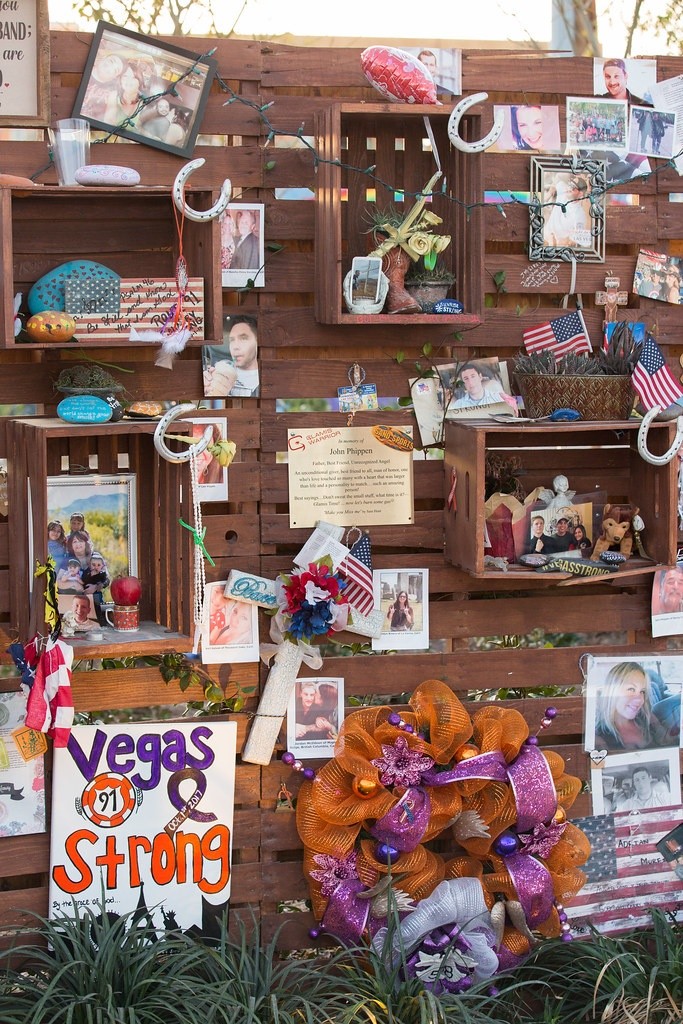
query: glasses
[{"left": 399, "top": 595, "right": 406, "bottom": 599}]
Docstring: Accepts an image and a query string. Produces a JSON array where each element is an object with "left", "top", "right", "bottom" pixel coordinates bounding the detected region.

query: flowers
[{"left": 275, "top": 554, "right": 355, "bottom": 650}]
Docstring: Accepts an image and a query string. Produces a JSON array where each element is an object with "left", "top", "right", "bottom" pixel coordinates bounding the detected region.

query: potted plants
[{"left": 405, "top": 258, "right": 455, "bottom": 314}]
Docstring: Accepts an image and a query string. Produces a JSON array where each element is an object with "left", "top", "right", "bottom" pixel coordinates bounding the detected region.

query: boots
[{"left": 367, "top": 229, "right": 422, "bottom": 314}]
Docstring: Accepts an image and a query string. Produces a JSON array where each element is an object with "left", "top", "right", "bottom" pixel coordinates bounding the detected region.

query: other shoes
[
  {"left": 652, "top": 149, "right": 660, "bottom": 155},
  {"left": 641, "top": 149, "right": 647, "bottom": 153}
]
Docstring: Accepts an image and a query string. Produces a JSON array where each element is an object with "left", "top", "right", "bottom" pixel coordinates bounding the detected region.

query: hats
[
  {"left": 557, "top": 514, "right": 570, "bottom": 523},
  {"left": 603, "top": 59, "right": 626, "bottom": 71}
]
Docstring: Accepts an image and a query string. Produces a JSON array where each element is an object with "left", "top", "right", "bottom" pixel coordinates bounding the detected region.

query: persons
[
  {"left": 47, "top": 512, "right": 111, "bottom": 632},
  {"left": 192, "top": 212, "right": 260, "bottom": 484},
  {"left": 596, "top": 564, "right": 683, "bottom": 812},
  {"left": 83, "top": 52, "right": 190, "bottom": 149},
  {"left": 295, "top": 681, "right": 341, "bottom": 740},
  {"left": 510, "top": 59, "right": 664, "bottom": 246},
  {"left": 547, "top": 475, "right": 578, "bottom": 510},
  {"left": 387, "top": 591, "right": 415, "bottom": 632},
  {"left": 436, "top": 367, "right": 504, "bottom": 409},
  {"left": 416, "top": 51, "right": 456, "bottom": 96},
  {"left": 527, "top": 514, "right": 593, "bottom": 553},
  {"left": 210, "top": 586, "right": 251, "bottom": 644},
  {"left": 634, "top": 259, "right": 683, "bottom": 306}
]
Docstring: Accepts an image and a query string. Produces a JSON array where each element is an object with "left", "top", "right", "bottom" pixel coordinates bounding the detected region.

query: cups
[
  {"left": 47, "top": 118, "right": 90, "bottom": 187},
  {"left": 105, "top": 604, "right": 140, "bottom": 632}
]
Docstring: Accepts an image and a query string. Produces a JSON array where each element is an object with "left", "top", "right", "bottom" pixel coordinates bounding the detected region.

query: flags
[
  {"left": 627, "top": 331, "right": 682, "bottom": 414},
  {"left": 334, "top": 531, "right": 374, "bottom": 616},
  {"left": 521, "top": 312, "right": 594, "bottom": 364}
]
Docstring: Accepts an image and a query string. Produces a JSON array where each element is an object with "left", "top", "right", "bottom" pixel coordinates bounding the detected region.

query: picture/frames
[
  {"left": 71, "top": 20, "right": 217, "bottom": 159},
  {"left": 26, "top": 472, "right": 142, "bottom": 612}
]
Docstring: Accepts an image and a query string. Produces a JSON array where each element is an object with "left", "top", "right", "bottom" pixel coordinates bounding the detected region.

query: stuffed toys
[{"left": 590, "top": 502, "right": 641, "bottom": 564}]
